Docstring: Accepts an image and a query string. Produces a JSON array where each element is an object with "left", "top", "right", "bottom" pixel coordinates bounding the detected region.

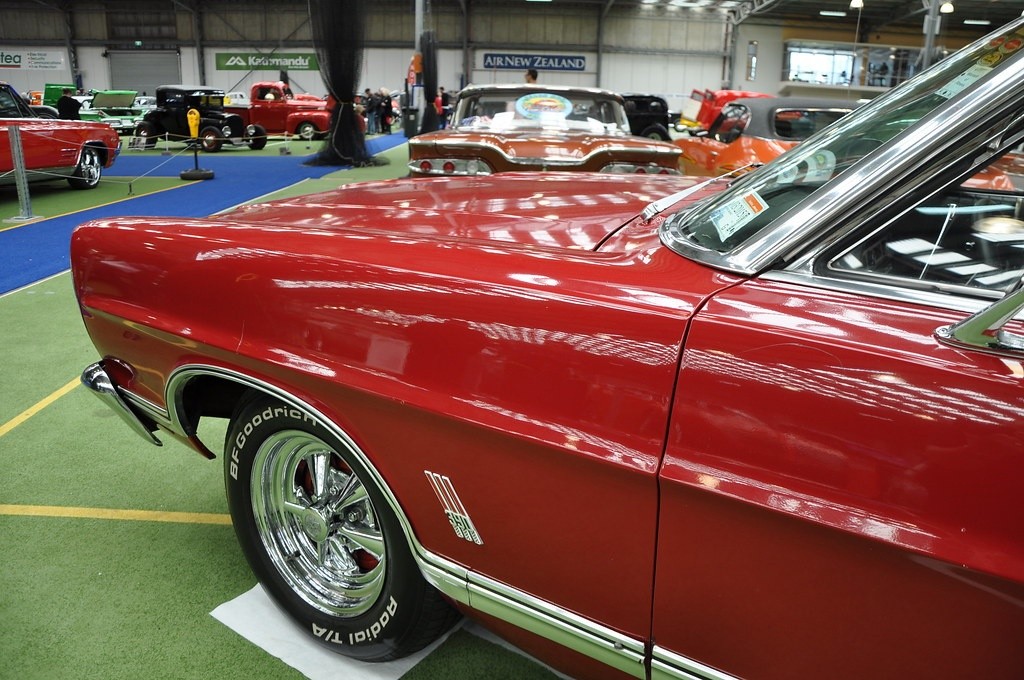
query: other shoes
[{"left": 365, "top": 129, "right": 392, "bottom": 135}]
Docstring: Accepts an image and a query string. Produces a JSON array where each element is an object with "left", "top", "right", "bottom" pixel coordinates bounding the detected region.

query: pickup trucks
[{"left": 222, "top": 80, "right": 333, "bottom": 141}]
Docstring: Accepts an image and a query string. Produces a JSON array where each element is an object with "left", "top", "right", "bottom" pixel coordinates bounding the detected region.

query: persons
[
  {"left": 440, "top": 87, "right": 448, "bottom": 130},
  {"left": 524, "top": 70, "right": 538, "bottom": 83},
  {"left": 365, "top": 88, "right": 392, "bottom": 134},
  {"left": 57, "top": 89, "right": 82, "bottom": 120},
  {"left": 22, "top": 92, "right": 31, "bottom": 104},
  {"left": 76, "top": 89, "right": 85, "bottom": 95}
]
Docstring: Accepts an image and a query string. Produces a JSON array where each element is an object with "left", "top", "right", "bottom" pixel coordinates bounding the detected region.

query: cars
[
  {"left": 674, "top": 95, "right": 1015, "bottom": 199},
  {"left": 23, "top": 80, "right": 404, "bottom": 139},
  {"left": 620, "top": 89, "right": 776, "bottom": 144},
  {"left": 409, "top": 81, "right": 683, "bottom": 177},
  {"left": 134, "top": 85, "right": 267, "bottom": 151},
  {"left": 0, "top": 79, "right": 123, "bottom": 191},
  {"left": 69, "top": 10, "right": 1024, "bottom": 680}
]
